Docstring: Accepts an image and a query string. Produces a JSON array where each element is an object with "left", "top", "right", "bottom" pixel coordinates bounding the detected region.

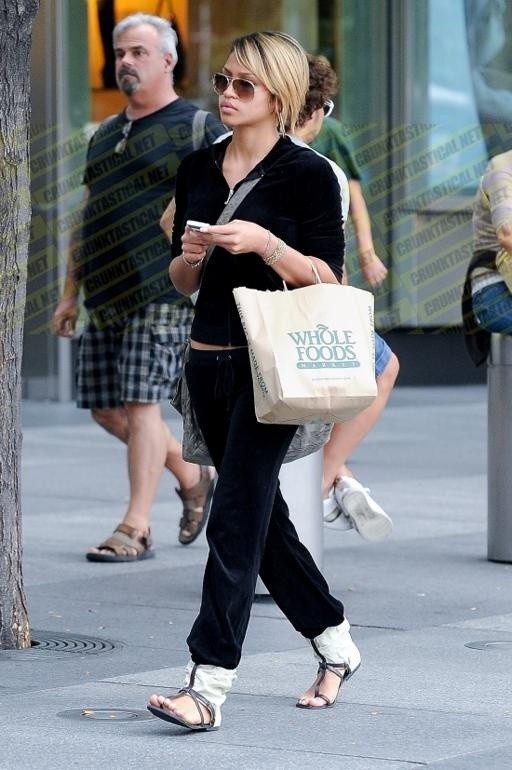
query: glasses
[
  {"left": 213, "top": 72, "right": 271, "bottom": 103},
  {"left": 322, "top": 99, "right": 335, "bottom": 118}
]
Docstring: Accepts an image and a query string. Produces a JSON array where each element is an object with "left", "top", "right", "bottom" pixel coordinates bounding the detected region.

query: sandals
[
  {"left": 148, "top": 686, "right": 223, "bottom": 732},
  {"left": 175, "top": 463, "right": 216, "bottom": 544},
  {"left": 296, "top": 651, "right": 362, "bottom": 710},
  {"left": 86, "top": 524, "right": 155, "bottom": 562}
]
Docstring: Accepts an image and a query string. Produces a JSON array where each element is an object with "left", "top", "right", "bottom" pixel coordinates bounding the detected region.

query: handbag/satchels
[
  {"left": 171, "top": 338, "right": 335, "bottom": 466},
  {"left": 232, "top": 284, "right": 377, "bottom": 425}
]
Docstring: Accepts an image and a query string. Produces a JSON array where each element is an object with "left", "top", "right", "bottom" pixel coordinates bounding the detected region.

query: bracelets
[
  {"left": 180, "top": 251, "right": 204, "bottom": 269},
  {"left": 260, "top": 228, "right": 271, "bottom": 256},
  {"left": 262, "top": 238, "right": 287, "bottom": 265}
]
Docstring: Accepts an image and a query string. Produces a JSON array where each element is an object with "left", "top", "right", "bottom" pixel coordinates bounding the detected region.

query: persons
[
  {"left": 465, "top": 148, "right": 511, "bottom": 337},
  {"left": 160, "top": 50, "right": 400, "bottom": 549},
  {"left": 142, "top": 28, "right": 366, "bottom": 733},
  {"left": 49, "top": 11, "right": 218, "bottom": 563},
  {"left": 300, "top": 108, "right": 393, "bottom": 291}
]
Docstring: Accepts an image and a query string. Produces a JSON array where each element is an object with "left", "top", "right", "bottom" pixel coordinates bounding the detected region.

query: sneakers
[{"left": 322, "top": 476, "right": 393, "bottom": 542}]
